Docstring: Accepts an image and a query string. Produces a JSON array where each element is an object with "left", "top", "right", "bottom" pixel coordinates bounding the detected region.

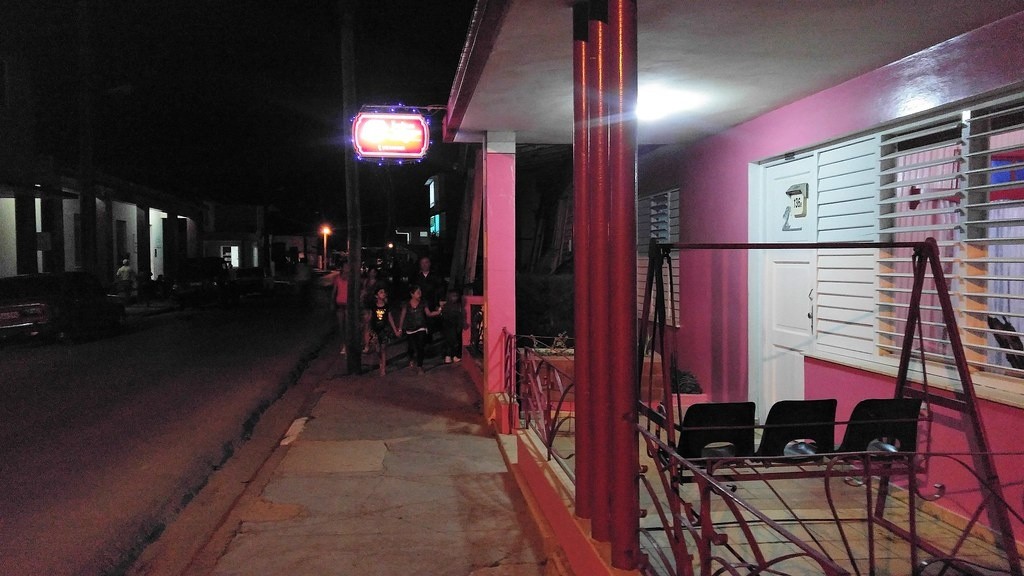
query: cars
[{"left": 0, "top": 269, "right": 127, "bottom": 345}]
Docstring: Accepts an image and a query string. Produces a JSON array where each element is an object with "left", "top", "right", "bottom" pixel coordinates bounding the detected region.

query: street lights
[{"left": 321, "top": 227, "right": 331, "bottom": 272}]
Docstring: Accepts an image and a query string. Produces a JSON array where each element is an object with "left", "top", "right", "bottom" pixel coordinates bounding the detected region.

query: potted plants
[{"left": 523, "top": 330, "right": 663, "bottom": 409}]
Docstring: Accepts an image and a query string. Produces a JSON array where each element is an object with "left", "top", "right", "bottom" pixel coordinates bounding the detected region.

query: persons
[
  {"left": 331, "top": 253, "right": 468, "bottom": 378},
  {"left": 296, "top": 258, "right": 312, "bottom": 298},
  {"left": 117, "top": 259, "right": 167, "bottom": 309}
]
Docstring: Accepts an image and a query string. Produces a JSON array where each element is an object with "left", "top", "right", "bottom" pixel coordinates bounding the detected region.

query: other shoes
[
  {"left": 409, "top": 360, "right": 416, "bottom": 370},
  {"left": 363, "top": 346, "right": 372, "bottom": 354},
  {"left": 340, "top": 344, "right": 347, "bottom": 354},
  {"left": 417, "top": 366, "right": 424, "bottom": 377},
  {"left": 380, "top": 362, "right": 386, "bottom": 377},
  {"left": 453, "top": 356, "right": 461, "bottom": 363},
  {"left": 445, "top": 356, "right": 452, "bottom": 364}
]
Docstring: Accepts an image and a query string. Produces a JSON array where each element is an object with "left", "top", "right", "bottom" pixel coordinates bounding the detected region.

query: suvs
[{"left": 170, "top": 257, "right": 234, "bottom": 305}]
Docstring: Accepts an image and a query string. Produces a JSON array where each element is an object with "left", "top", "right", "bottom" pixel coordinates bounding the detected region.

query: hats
[{"left": 123, "top": 259, "right": 130, "bottom": 265}]
